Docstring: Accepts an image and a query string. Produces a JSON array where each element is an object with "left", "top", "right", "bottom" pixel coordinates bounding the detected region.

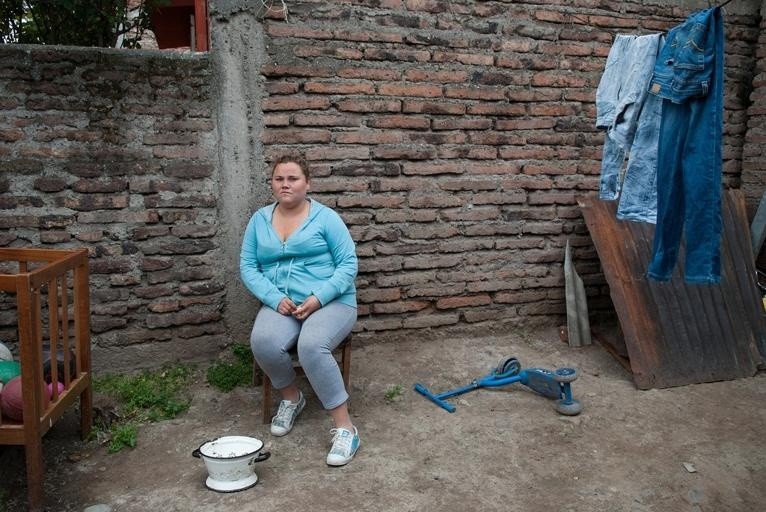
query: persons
[{"left": 238, "top": 151, "right": 361, "bottom": 468}]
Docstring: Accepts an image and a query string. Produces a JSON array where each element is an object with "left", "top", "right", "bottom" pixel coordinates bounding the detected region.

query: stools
[{"left": 252, "top": 332, "right": 352, "bottom": 424}]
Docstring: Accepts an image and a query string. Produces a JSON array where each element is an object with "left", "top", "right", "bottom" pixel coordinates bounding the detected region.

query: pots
[{"left": 191, "top": 436, "right": 271, "bottom": 492}]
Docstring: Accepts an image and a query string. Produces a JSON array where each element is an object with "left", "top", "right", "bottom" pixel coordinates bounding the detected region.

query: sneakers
[
  {"left": 326, "top": 425, "right": 361, "bottom": 467},
  {"left": 270, "top": 390, "right": 307, "bottom": 437}
]
